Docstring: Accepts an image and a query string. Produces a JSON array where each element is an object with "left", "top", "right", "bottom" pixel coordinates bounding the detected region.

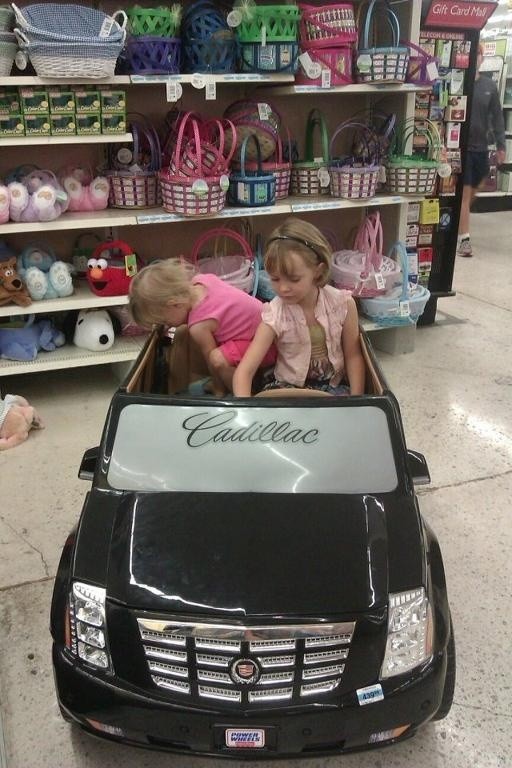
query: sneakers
[{"left": 458, "top": 237, "right": 473, "bottom": 257}]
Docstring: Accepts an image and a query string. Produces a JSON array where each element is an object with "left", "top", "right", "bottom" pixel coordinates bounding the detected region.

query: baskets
[
  {"left": 99, "top": 97, "right": 442, "bottom": 216},
  {"left": 0, "top": 0, "right": 410, "bottom": 82},
  {"left": 192, "top": 211, "right": 431, "bottom": 328}
]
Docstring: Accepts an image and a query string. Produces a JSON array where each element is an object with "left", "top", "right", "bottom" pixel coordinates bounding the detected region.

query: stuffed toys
[
  {"left": 1, "top": 393, "right": 41, "bottom": 448},
  {"left": 0, "top": 165, "right": 116, "bottom": 362}
]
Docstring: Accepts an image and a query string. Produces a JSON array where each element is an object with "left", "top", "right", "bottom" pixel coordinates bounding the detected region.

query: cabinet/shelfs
[
  {"left": 475, "top": 61, "right": 512, "bottom": 212},
  {"left": 0, "top": 73, "right": 428, "bottom": 382}
]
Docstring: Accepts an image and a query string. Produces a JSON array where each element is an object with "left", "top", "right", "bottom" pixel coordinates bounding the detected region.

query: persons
[
  {"left": 457, "top": 45, "right": 506, "bottom": 257},
  {"left": 126, "top": 253, "right": 277, "bottom": 403},
  {"left": 233, "top": 219, "right": 369, "bottom": 398}
]
